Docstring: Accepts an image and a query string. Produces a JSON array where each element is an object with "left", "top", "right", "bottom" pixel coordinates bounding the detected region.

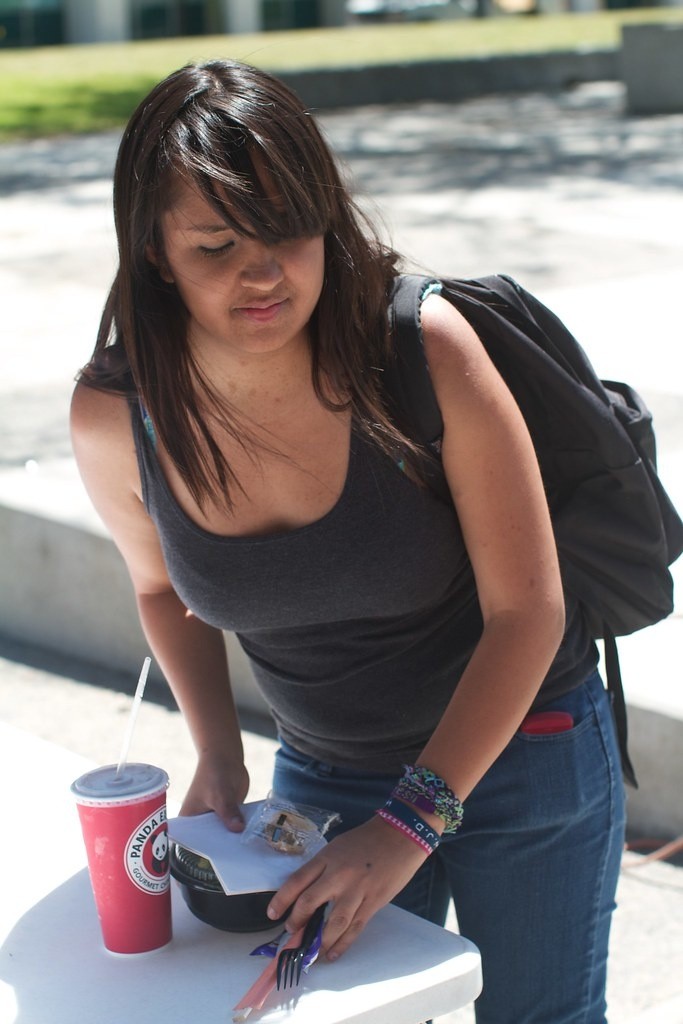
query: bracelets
[{"left": 378, "top": 764, "right": 464, "bottom": 855}]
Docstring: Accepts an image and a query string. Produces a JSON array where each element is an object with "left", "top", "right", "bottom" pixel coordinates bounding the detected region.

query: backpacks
[{"left": 392, "top": 273, "right": 683, "bottom": 789}]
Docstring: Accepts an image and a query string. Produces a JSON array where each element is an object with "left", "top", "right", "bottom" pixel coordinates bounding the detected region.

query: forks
[{"left": 276, "top": 901, "right": 329, "bottom": 990}]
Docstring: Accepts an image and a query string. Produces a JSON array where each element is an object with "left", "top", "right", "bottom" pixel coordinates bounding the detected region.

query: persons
[{"left": 66, "top": 60, "right": 627, "bottom": 1024}]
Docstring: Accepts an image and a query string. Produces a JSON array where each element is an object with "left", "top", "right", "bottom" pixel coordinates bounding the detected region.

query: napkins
[{"left": 166, "top": 799, "right": 329, "bottom": 896}]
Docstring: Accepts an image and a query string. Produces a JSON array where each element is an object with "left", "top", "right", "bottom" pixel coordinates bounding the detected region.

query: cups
[{"left": 70, "top": 763, "right": 172, "bottom": 954}]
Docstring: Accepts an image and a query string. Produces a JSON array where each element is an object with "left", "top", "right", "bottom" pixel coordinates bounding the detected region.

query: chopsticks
[{"left": 232, "top": 1007, "right": 252, "bottom": 1023}]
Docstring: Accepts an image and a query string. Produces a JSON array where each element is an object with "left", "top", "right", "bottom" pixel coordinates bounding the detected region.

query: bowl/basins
[{"left": 170, "top": 843, "right": 296, "bottom": 933}]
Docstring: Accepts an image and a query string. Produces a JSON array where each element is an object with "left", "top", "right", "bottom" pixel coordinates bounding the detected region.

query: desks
[{"left": 0, "top": 752, "right": 482, "bottom": 1024}]
